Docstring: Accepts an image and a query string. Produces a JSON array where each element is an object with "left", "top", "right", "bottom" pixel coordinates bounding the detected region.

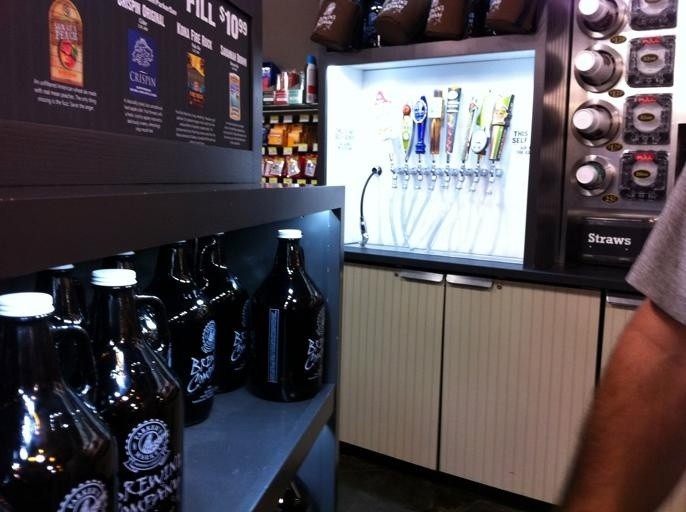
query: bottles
[
  {"left": 304, "top": 54, "right": 316, "bottom": 104},
  {"left": 1, "top": 229, "right": 247, "bottom": 510},
  {"left": 248, "top": 229, "right": 326, "bottom": 402}
]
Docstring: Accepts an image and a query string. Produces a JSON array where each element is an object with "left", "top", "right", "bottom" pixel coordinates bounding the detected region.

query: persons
[{"left": 550, "top": 160, "right": 686, "bottom": 512}]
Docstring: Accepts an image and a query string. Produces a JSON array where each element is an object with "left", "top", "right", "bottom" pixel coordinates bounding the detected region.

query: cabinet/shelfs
[
  {"left": 601, "top": 290, "right": 685, "bottom": 509},
  {"left": 262, "top": 103, "right": 323, "bottom": 185},
  {"left": 0, "top": 177, "right": 342, "bottom": 511},
  {"left": 340, "top": 260, "right": 600, "bottom": 506}
]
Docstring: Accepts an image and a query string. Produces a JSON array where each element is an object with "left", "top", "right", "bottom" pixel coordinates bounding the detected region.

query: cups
[
  {"left": 577, "top": 0, "right": 615, "bottom": 32},
  {"left": 574, "top": 49, "right": 614, "bottom": 86},
  {"left": 572, "top": 106, "right": 613, "bottom": 140},
  {"left": 575, "top": 163, "right": 604, "bottom": 188}
]
